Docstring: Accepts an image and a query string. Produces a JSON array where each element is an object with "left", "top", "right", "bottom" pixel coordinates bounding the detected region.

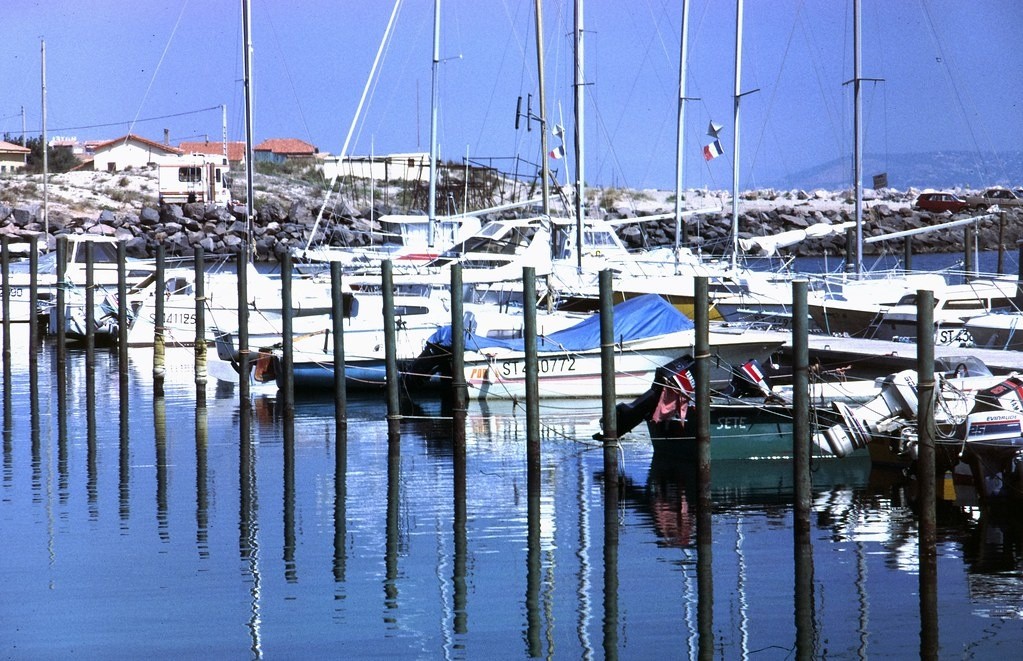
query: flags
[
  {"left": 673, "top": 369, "right": 695, "bottom": 392},
  {"left": 704, "top": 139, "right": 724, "bottom": 161},
  {"left": 742, "top": 359, "right": 766, "bottom": 383},
  {"left": 548, "top": 145, "right": 567, "bottom": 159}
]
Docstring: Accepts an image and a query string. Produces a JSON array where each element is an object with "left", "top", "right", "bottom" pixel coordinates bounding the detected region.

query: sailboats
[{"left": 0, "top": 1, "right": 1022, "bottom": 513}]
